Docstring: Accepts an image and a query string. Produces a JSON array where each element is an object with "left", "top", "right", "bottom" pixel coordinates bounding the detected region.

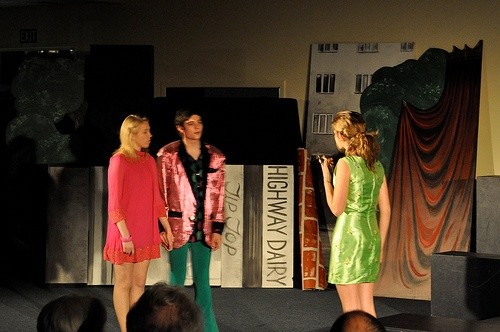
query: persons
[
  {"left": 330, "top": 310, "right": 386, "bottom": 332},
  {"left": 37, "top": 294, "right": 107, "bottom": 332},
  {"left": 126, "top": 282, "right": 203, "bottom": 332},
  {"left": 103, "top": 115, "right": 174, "bottom": 332},
  {"left": 317, "top": 111, "right": 391, "bottom": 318},
  {"left": 157, "top": 111, "right": 226, "bottom": 332}
]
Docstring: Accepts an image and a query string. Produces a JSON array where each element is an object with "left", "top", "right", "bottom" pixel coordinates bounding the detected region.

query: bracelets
[
  {"left": 121, "top": 235, "right": 132, "bottom": 242},
  {"left": 212, "top": 231, "right": 222, "bottom": 234}
]
